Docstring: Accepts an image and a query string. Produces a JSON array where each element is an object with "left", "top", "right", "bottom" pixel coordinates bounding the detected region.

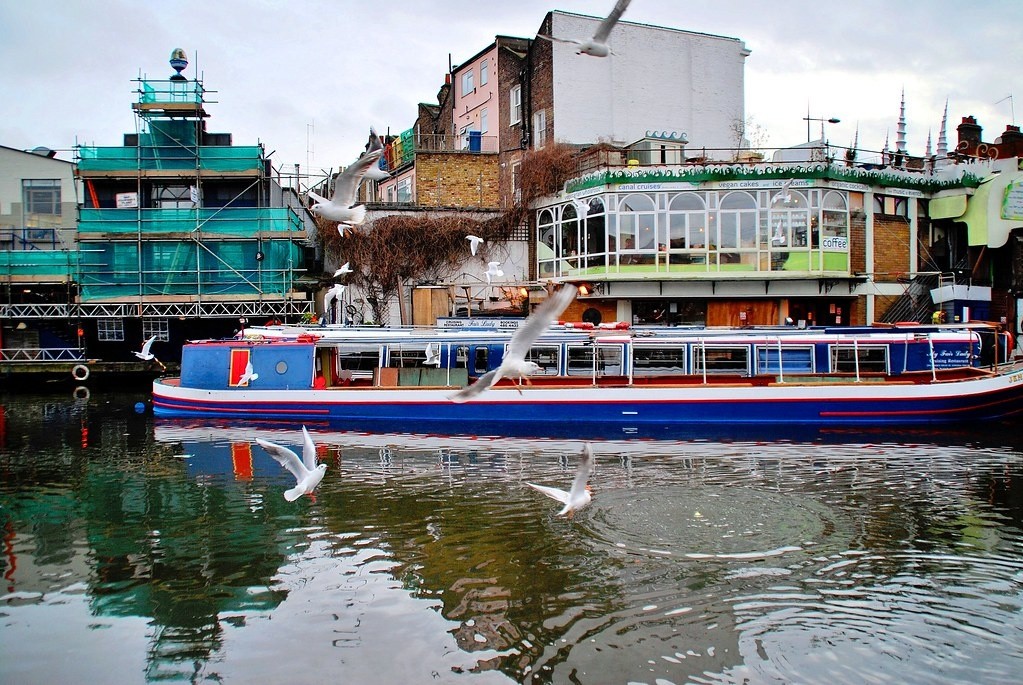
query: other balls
[
  {"left": 135, "top": 409, "right": 145, "bottom": 413},
  {"left": 135, "top": 402, "right": 144, "bottom": 409}
]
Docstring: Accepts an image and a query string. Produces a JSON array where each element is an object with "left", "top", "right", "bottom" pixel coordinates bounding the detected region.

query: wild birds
[
  {"left": 465, "top": 235, "right": 484, "bottom": 257},
  {"left": 481, "top": 262, "right": 504, "bottom": 285},
  {"left": 332, "top": 261, "right": 353, "bottom": 278},
  {"left": 453, "top": 282, "right": 579, "bottom": 402},
  {"left": 358, "top": 125, "right": 393, "bottom": 189},
  {"left": 307, "top": 148, "right": 382, "bottom": 237},
  {"left": 254, "top": 425, "right": 331, "bottom": 504},
  {"left": 536, "top": 0, "right": 632, "bottom": 58},
  {"left": 422, "top": 342, "right": 442, "bottom": 366},
  {"left": 130, "top": 335, "right": 157, "bottom": 361},
  {"left": 323, "top": 284, "right": 346, "bottom": 314},
  {"left": 524, "top": 443, "right": 593, "bottom": 520}
]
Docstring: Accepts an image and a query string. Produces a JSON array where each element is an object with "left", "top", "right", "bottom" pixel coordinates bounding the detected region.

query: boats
[
  {"left": 140, "top": 413, "right": 1021, "bottom": 505},
  {"left": 150, "top": 293, "right": 1022, "bottom": 426}
]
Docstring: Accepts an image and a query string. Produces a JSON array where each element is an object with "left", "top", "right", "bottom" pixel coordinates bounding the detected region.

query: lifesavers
[
  {"left": 549, "top": 320, "right": 566, "bottom": 325},
  {"left": 598, "top": 320, "right": 631, "bottom": 331},
  {"left": 71, "top": 364, "right": 90, "bottom": 381},
  {"left": 72, "top": 386, "right": 90, "bottom": 401},
  {"left": 564, "top": 321, "right": 596, "bottom": 330},
  {"left": 1003, "top": 331, "right": 1014, "bottom": 362},
  {"left": 265, "top": 319, "right": 282, "bottom": 327}
]
